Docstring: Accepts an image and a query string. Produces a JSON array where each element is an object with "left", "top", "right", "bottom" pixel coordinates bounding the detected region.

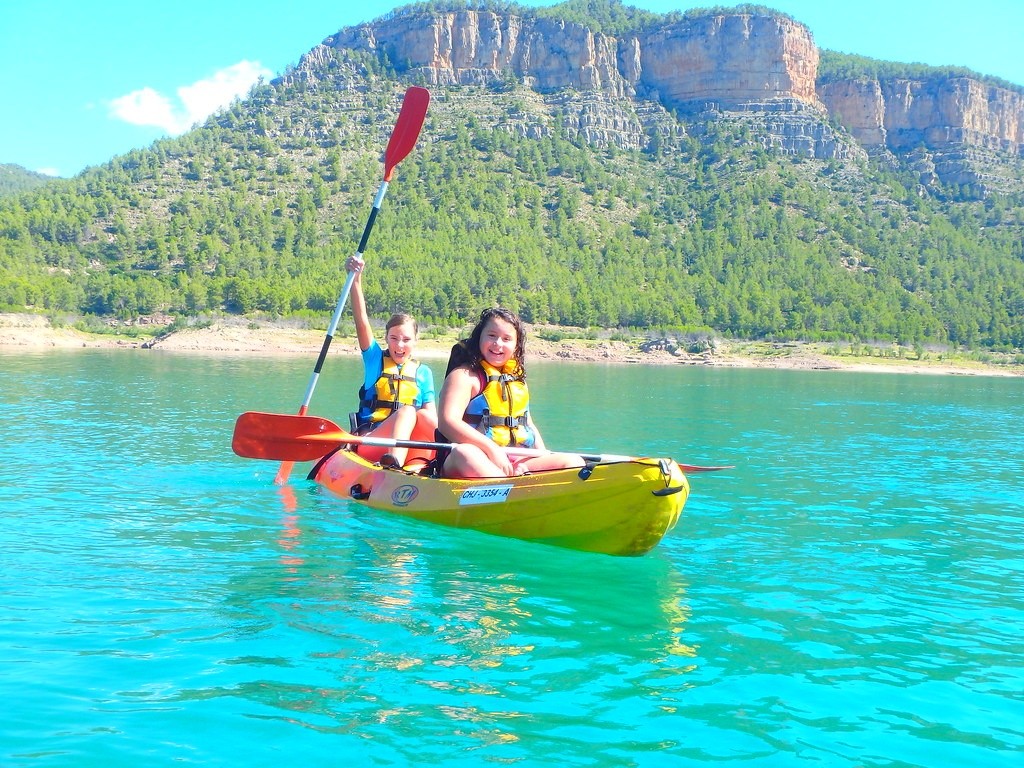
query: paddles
[
  {"left": 274, "top": 86, "right": 433, "bottom": 485},
  {"left": 230, "top": 411, "right": 732, "bottom": 473}
]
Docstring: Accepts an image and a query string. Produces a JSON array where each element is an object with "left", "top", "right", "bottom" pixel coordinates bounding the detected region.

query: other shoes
[{"left": 380, "top": 453, "right": 401, "bottom": 470}]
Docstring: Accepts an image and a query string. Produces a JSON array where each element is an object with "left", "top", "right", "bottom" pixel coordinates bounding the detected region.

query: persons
[
  {"left": 345, "top": 254, "right": 438, "bottom": 470},
  {"left": 438, "top": 307, "right": 586, "bottom": 480}
]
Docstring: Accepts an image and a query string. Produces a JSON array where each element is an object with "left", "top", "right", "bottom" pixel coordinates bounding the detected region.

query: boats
[{"left": 311, "top": 412, "right": 690, "bottom": 557}]
